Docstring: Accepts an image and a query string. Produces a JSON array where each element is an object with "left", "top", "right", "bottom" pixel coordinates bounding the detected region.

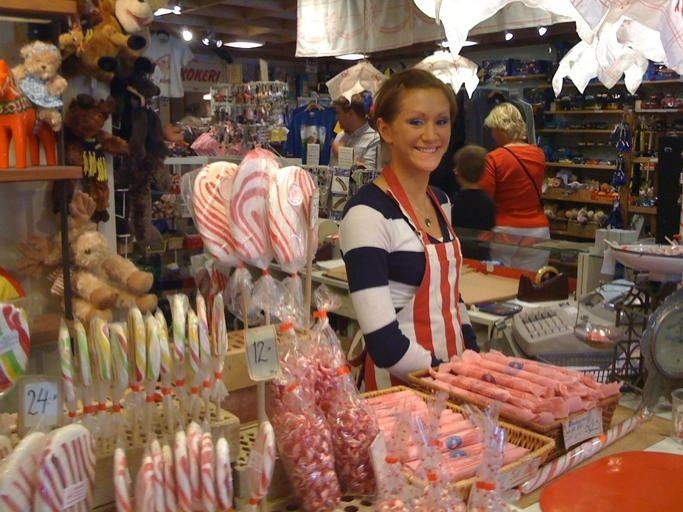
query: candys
[{"left": 0, "top": 146, "right": 319, "bottom": 512}]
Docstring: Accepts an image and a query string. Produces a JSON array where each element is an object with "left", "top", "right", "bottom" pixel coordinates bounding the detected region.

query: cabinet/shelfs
[
  {"left": 462, "top": 74, "right": 683, "bottom": 243},
  {"left": 0, "top": 0, "right": 83, "bottom": 375},
  {"left": 115, "top": 156, "right": 383, "bottom": 333}
]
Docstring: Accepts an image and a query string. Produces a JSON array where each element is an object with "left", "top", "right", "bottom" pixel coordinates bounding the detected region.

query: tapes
[{"left": 536, "top": 265, "right": 558, "bottom": 285}]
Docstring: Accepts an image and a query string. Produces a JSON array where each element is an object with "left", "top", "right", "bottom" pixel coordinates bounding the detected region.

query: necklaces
[{"left": 410, "top": 193, "right": 433, "bottom": 227}]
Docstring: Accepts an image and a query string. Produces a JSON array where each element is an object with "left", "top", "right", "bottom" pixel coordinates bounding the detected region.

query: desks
[{"left": 230, "top": 258, "right": 577, "bottom": 401}]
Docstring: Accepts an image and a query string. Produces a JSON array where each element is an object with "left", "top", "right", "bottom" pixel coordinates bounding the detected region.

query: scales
[{"left": 611, "top": 244, "right": 683, "bottom": 415}]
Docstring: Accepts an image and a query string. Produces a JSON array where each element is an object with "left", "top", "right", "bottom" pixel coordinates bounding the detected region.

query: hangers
[
  {"left": 155, "top": 22, "right": 170, "bottom": 40},
  {"left": 304, "top": 91, "right": 328, "bottom": 114}
]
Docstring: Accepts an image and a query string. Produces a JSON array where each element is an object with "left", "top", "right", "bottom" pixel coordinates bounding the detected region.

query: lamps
[
  {"left": 201, "top": 29, "right": 223, "bottom": 49},
  {"left": 504, "top": 29, "right": 514, "bottom": 42},
  {"left": 178, "top": 25, "right": 193, "bottom": 42},
  {"left": 535, "top": 24, "right": 548, "bottom": 36},
  {"left": 224, "top": 36, "right": 266, "bottom": 49}
]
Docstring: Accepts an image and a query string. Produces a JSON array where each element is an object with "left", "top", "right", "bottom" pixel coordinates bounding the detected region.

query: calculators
[{"left": 480, "top": 302, "right": 522, "bottom": 316}]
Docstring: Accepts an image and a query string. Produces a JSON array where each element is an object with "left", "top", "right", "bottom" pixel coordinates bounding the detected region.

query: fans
[{"left": 152, "top": 0, "right": 183, "bottom": 16}]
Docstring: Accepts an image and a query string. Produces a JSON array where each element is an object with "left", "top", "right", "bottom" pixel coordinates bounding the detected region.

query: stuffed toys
[
  {"left": 9, "top": 0, "right": 166, "bottom": 323},
  {"left": 544, "top": 199, "right": 609, "bottom": 227},
  {"left": 542, "top": 171, "right": 615, "bottom": 195},
  {"left": 10, "top": 0, "right": 166, "bottom": 330}
]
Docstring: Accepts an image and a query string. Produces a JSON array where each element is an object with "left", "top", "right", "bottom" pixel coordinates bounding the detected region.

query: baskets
[
  {"left": 361, "top": 384, "right": 555, "bottom": 502},
  {"left": 408, "top": 364, "right": 621, "bottom": 463}
]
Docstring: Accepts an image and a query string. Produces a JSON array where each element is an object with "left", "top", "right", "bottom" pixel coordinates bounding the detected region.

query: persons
[
  {"left": 327, "top": 89, "right": 384, "bottom": 222},
  {"left": 450, "top": 145, "right": 495, "bottom": 261},
  {"left": 338, "top": 68, "right": 480, "bottom": 395},
  {"left": 481, "top": 102, "right": 551, "bottom": 280}
]
